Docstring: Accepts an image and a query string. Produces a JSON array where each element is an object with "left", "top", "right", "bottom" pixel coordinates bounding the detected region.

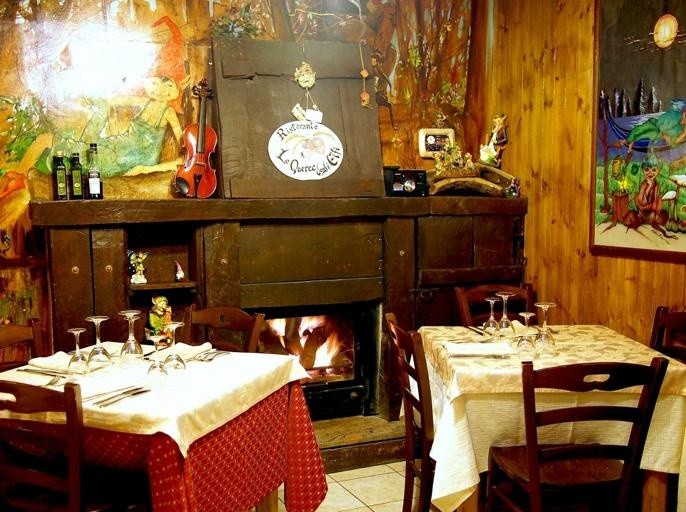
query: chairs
[
  {"left": 383, "top": 312, "right": 437, "bottom": 512},
  {"left": 0, "top": 377, "right": 117, "bottom": 512},
  {"left": 484, "top": 357, "right": 670, "bottom": 512},
  {"left": 180, "top": 304, "right": 266, "bottom": 352},
  {"left": 454, "top": 282, "right": 537, "bottom": 329},
  {"left": 648, "top": 305, "right": 686, "bottom": 368}
]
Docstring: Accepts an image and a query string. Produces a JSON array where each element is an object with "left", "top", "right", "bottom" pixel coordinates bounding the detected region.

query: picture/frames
[{"left": 586, "top": 0, "right": 685, "bottom": 265}]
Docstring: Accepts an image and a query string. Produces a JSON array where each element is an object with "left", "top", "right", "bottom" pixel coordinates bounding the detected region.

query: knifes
[
  {"left": 450, "top": 353, "right": 512, "bottom": 361},
  {"left": 464, "top": 323, "right": 487, "bottom": 336},
  {"left": 93, "top": 386, "right": 142, "bottom": 404},
  {"left": 17, "top": 368, "right": 64, "bottom": 378},
  {"left": 95, "top": 387, "right": 154, "bottom": 408}
]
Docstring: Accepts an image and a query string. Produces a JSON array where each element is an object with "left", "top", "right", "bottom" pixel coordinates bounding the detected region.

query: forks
[
  {"left": 464, "top": 339, "right": 495, "bottom": 348},
  {"left": 191, "top": 344, "right": 233, "bottom": 361},
  {"left": 48, "top": 378, "right": 77, "bottom": 389},
  {"left": 200, "top": 351, "right": 218, "bottom": 362},
  {"left": 36, "top": 374, "right": 60, "bottom": 388}
]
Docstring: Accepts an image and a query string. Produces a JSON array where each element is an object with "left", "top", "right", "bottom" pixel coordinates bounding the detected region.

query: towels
[
  {"left": 148, "top": 342, "right": 211, "bottom": 363},
  {"left": 27, "top": 350, "right": 105, "bottom": 375},
  {"left": 446, "top": 341, "right": 518, "bottom": 356},
  {"left": 53, "top": 375, "right": 137, "bottom": 401}
]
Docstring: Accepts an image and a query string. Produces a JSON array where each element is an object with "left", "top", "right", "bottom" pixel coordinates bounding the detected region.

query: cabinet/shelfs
[{"left": 27, "top": 193, "right": 532, "bottom": 476}]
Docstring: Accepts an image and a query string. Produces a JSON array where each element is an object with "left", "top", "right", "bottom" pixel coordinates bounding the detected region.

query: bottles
[
  {"left": 52, "top": 151, "right": 67, "bottom": 201},
  {"left": 69, "top": 153, "right": 84, "bottom": 200},
  {"left": 86, "top": 143, "right": 104, "bottom": 200}
]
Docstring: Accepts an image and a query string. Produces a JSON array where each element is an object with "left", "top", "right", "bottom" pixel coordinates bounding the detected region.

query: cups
[{"left": 517, "top": 312, "right": 536, "bottom": 349}]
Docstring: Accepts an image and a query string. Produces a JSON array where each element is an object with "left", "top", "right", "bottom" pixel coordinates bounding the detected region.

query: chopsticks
[
  {"left": 81, "top": 385, "right": 134, "bottom": 404},
  {"left": 143, "top": 345, "right": 171, "bottom": 358}
]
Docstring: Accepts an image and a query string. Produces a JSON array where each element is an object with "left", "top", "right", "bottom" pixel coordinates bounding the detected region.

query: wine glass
[
  {"left": 161, "top": 321, "right": 186, "bottom": 373},
  {"left": 483, "top": 297, "right": 501, "bottom": 333},
  {"left": 119, "top": 311, "right": 144, "bottom": 358},
  {"left": 85, "top": 315, "right": 111, "bottom": 369},
  {"left": 534, "top": 303, "right": 557, "bottom": 347},
  {"left": 67, "top": 328, "right": 88, "bottom": 373},
  {"left": 495, "top": 291, "right": 516, "bottom": 337}
]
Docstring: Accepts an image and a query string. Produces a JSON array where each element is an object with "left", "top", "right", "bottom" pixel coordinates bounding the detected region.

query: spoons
[{"left": 207, "top": 351, "right": 226, "bottom": 361}]
